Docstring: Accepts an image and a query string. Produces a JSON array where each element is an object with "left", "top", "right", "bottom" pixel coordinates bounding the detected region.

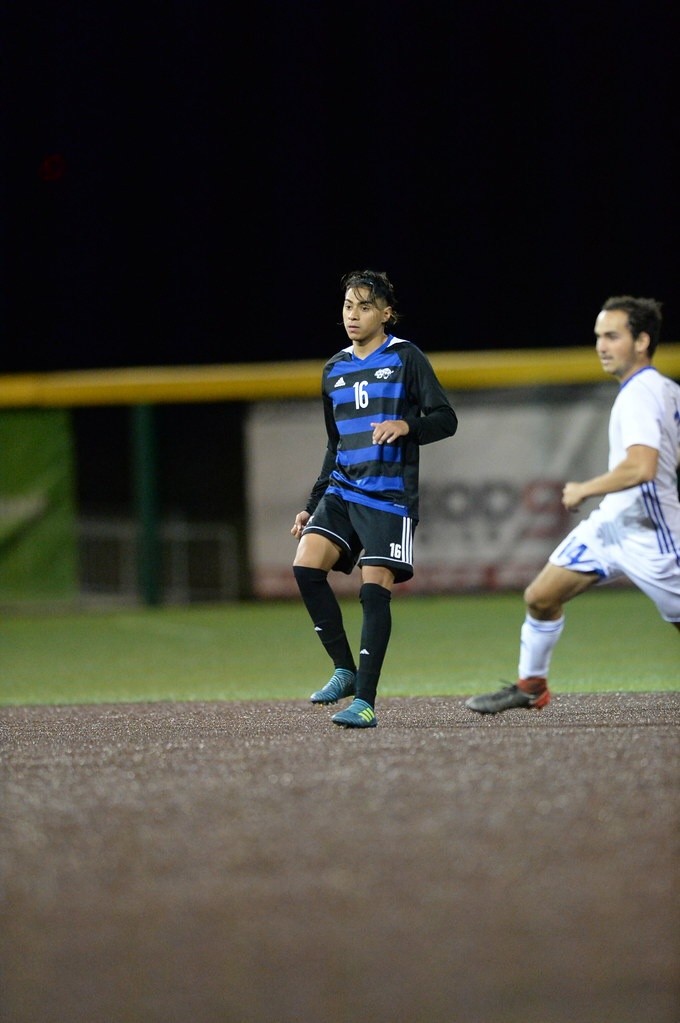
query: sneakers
[
  {"left": 465, "top": 679, "right": 550, "bottom": 716},
  {"left": 331, "top": 699, "right": 377, "bottom": 729},
  {"left": 310, "top": 667, "right": 359, "bottom": 705}
]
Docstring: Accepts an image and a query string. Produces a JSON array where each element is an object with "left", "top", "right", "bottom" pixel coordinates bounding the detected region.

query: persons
[
  {"left": 464, "top": 296, "right": 680, "bottom": 715},
  {"left": 292, "top": 271, "right": 458, "bottom": 729}
]
